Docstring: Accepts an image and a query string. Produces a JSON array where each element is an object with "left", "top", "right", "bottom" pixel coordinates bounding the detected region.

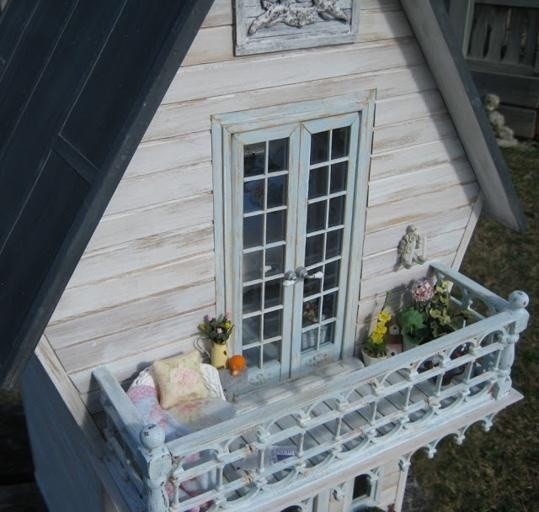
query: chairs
[{"left": 125, "top": 363, "right": 228, "bottom": 511}]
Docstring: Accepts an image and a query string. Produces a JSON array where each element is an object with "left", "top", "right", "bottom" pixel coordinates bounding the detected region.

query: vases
[
  {"left": 302, "top": 324, "right": 327, "bottom": 349},
  {"left": 210, "top": 340, "right": 228, "bottom": 369},
  {"left": 401, "top": 331, "right": 423, "bottom": 352},
  {"left": 361, "top": 345, "right": 391, "bottom": 366}
]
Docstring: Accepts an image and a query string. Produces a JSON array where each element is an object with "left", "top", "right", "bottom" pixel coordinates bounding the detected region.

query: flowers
[
  {"left": 301, "top": 298, "right": 329, "bottom": 327},
  {"left": 397, "top": 279, "right": 437, "bottom": 344},
  {"left": 195, "top": 311, "right": 236, "bottom": 350},
  {"left": 364, "top": 289, "right": 396, "bottom": 355}
]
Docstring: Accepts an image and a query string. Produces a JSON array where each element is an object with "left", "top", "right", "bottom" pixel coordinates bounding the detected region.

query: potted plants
[{"left": 425, "top": 279, "right": 475, "bottom": 385}]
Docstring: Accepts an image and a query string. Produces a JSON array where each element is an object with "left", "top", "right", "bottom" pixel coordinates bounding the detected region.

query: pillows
[{"left": 149, "top": 350, "right": 210, "bottom": 410}]
